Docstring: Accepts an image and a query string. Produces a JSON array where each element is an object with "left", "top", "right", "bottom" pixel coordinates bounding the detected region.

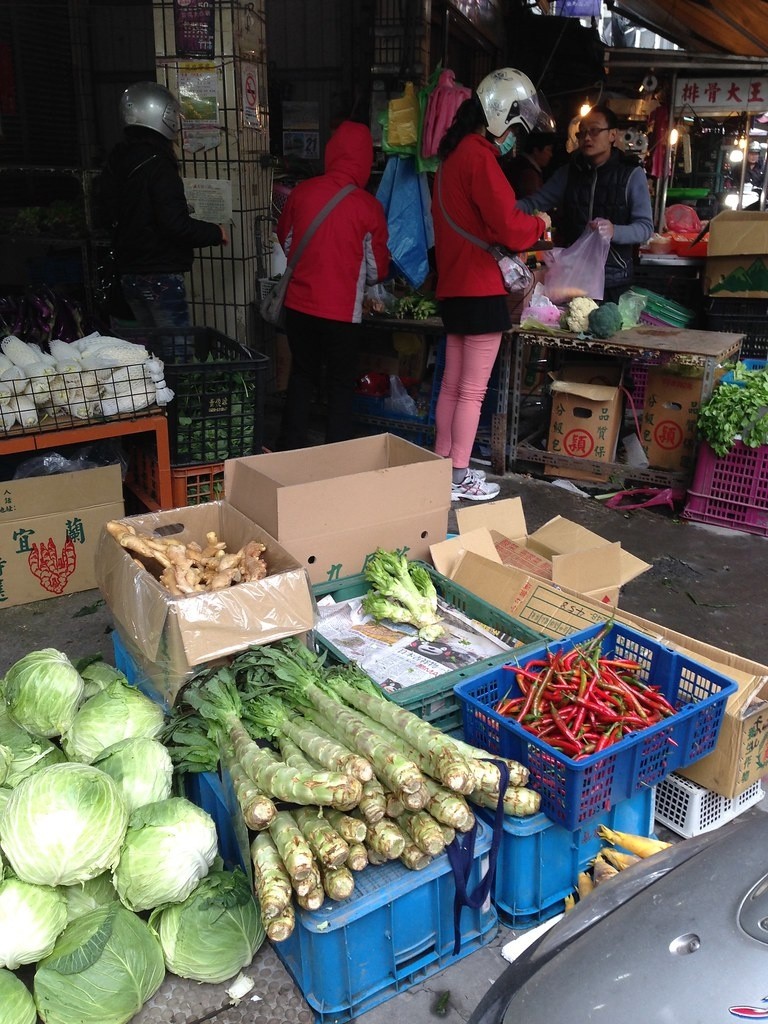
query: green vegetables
[
  {"left": 698, "top": 361, "right": 768, "bottom": 457},
  {"left": 391, "top": 294, "right": 439, "bottom": 320},
  {"left": 174, "top": 354, "right": 255, "bottom": 506}
]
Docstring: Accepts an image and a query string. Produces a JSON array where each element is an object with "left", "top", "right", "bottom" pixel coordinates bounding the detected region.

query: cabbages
[{"left": 0, "top": 649, "right": 266, "bottom": 1024}]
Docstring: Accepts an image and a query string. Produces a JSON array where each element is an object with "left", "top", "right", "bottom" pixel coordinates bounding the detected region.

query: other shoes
[{"left": 261, "top": 437, "right": 273, "bottom": 455}]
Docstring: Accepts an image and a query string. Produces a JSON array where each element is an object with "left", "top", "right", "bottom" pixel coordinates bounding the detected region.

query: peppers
[{"left": 476, "top": 608, "right": 712, "bottom": 821}]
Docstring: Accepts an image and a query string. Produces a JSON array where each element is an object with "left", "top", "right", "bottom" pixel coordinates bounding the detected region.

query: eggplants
[{"left": 0, "top": 283, "right": 89, "bottom": 354}]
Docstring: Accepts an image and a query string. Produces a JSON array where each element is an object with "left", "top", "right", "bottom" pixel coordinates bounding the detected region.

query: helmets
[
  {"left": 476, "top": 68, "right": 543, "bottom": 139},
  {"left": 748, "top": 140, "right": 762, "bottom": 152},
  {"left": 122, "top": 82, "right": 184, "bottom": 148}
]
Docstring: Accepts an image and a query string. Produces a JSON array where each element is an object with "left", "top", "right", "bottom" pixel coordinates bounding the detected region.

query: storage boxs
[
  {"left": 606, "top": 98, "right": 662, "bottom": 121},
  {"left": 504, "top": 267, "right": 548, "bottom": 326},
  {"left": 702, "top": 211, "right": 768, "bottom": 296},
  {"left": 0, "top": 463, "right": 130, "bottom": 611},
  {"left": 672, "top": 230, "right": 708, "bottom": 256},
  {"left": 634, "top": 243, "right": 705, "bottom": 313},
  {"left": 88, "top": 284, "right": 768, "bottom": 1024},
  {"left": 272, "top": 178, "right": 291, "bottom": 232}
]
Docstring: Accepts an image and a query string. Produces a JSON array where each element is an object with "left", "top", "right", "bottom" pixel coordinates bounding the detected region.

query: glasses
[{"left": 574, "top": 126, "right": 613, "bottom": 139}]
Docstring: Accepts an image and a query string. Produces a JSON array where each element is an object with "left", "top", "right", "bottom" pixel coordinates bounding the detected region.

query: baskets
[
  {"left": 273, "top": 182, "right": 292, "bottom": 222},
  {"left": 629, "top": 285, "right": 695, "bottom": 327}
]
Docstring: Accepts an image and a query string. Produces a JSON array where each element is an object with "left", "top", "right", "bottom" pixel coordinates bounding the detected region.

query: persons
[
  {"left": 261, "top": 120, "right": 389, "bottom": 453},
  {"left": 725, "top": 141, "right": 766, "bottom": 211},
  {"left": 515, "top": 132, "right": 555, "bottom": 201},
  {"left": 435, "top": 67, "right": 551, "bottom": 502},
  {"left": 101, "top": 81, "right": 228, "bottom": 355},
  {"left": 515, "top": 104, "right": 653, "bottom": 305}
]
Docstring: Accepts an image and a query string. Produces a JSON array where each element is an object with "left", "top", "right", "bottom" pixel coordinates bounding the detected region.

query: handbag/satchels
[
  {"left": 666, "top": 203, "right": 703, "bottom": 234},
  {"left": 93, "top": 250, "right": 138, "bottom": 322},
  {"left": 544, "top": 220, "right": 611, "bottom": 301},
  {"left": 488, "top": 244, "right": 533, "bottom": 292},
  {"left": 261, "top": 268, "right": 294, "bottom": 332}
]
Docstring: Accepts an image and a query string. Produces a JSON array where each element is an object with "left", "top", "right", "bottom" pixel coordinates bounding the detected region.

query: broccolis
[
  {"left": 588, "top": 303, "right": 624, "bottom": 339},
  {"left": 566, "top": 297, "right": 599, "bottom": 332}
]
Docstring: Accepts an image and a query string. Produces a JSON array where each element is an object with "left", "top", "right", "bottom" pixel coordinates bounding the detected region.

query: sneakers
[{"left": 451, "top": 467, "right": 501, "bottom": 501}]
